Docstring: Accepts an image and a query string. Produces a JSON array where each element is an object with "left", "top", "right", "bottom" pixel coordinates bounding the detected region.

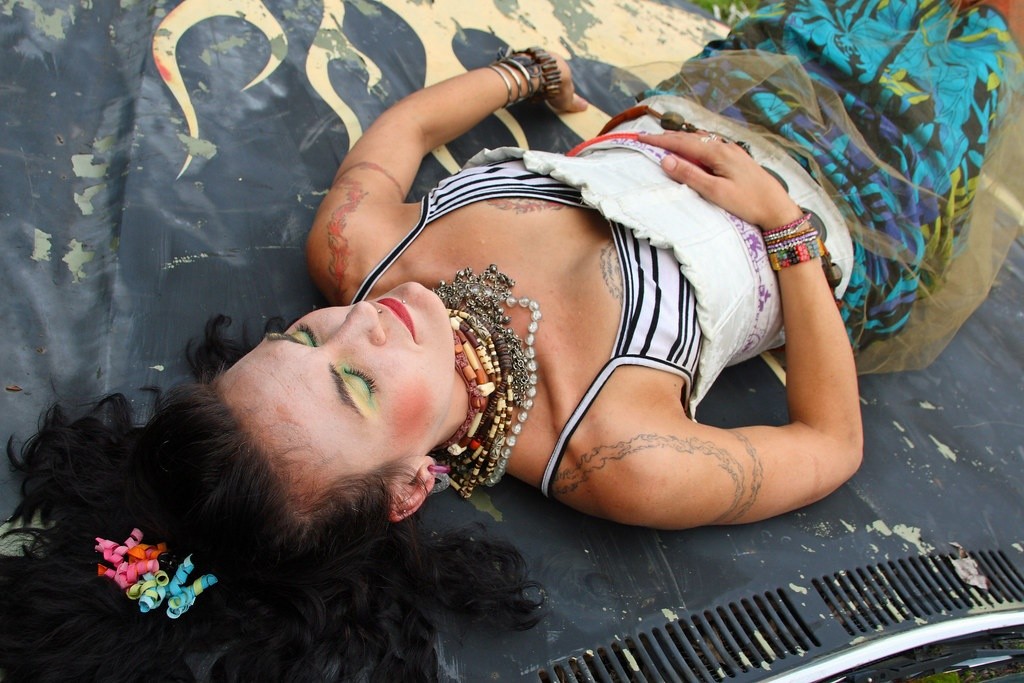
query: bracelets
[
  {"left": 763, "top": 213, "right": 825, "bottom": 270},
  {"left": 491, "top": 45, "right": 561, "bottom": 107}
]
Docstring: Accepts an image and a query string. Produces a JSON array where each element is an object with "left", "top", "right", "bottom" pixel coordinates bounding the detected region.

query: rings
[
  {"left": 700, "top": 137, "right": 709, "bottom": 142},
  {"left": 709, "top": 134, "right": 718, "bottom": 141}
]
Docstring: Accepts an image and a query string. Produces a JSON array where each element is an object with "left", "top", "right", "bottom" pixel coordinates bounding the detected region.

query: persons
[{"left": 0, "top": 0, "right": 1024, "bottom": 683}]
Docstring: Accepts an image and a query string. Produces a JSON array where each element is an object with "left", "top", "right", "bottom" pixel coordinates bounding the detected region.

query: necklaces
[{"left": 433, "top": 265, "right": 542, "bottom": 500}]
